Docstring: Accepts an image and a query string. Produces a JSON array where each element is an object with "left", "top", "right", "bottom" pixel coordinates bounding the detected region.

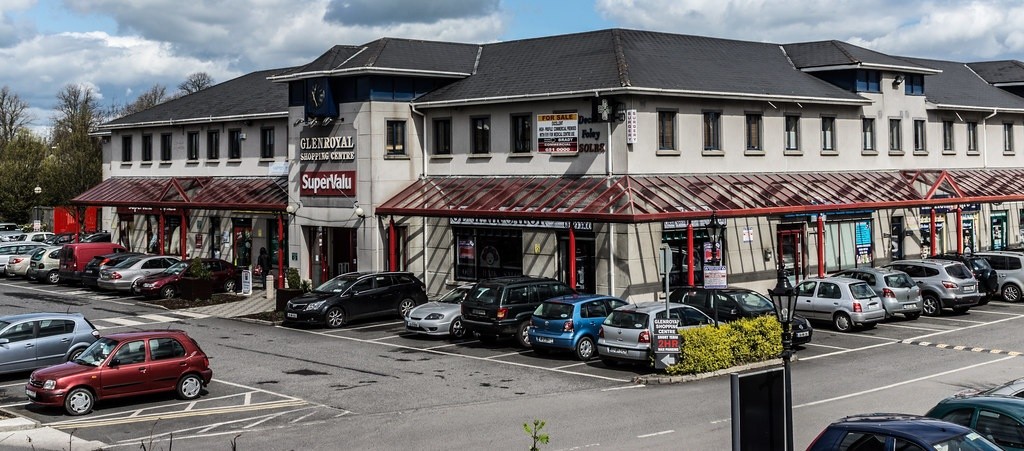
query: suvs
[
  {"left": 460, "top": 275, "right": 578, "bottom": 348},
  {"left": 284, "top": 270, "right": 428, "bottom": 329},
  {"left": 924, "top": 253, "right": 998, "bottom": 306},
  {"left": 806, "top": 394, "right": 1024, "bottom": 451},
  {"left": 878, "top": 259, "right": 980, "bottom": 316},
  {"left": 971, "top": 251, "right": 1024, "bottom": 302},
  {"left": 669, "top": 286, "right": 813, "bottom": 346},
  {"left": 25, "top": 330, "right": 212, "bottom": 417}
]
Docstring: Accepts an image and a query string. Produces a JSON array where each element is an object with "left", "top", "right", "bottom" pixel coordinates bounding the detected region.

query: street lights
[
  {"left": 767, "top": 250, "right": 799, "bottom": 451},
  {"left": 34, "top": 184, "right": 41, "bottom": 220},
  {"left": 703, "top": 209, "right": 725, "bottom": 331}
]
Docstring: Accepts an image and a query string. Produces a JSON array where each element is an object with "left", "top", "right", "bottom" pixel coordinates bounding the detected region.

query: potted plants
[
  {"left": 180, "top": 256, "right": 213, "bottom": 301},
  {"left": 276, "top": 266, "right": 306, "bottom": 312}
]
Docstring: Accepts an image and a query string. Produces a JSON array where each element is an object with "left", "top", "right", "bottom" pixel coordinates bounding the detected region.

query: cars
[
  {"left": 597, "top": 302, "right": 720, "bottom": 367},
  {"left": 830, "top": 268, "right": 923, "bottom": 322},
  {"left": 132, "top": 258, "right": 242, "bottom": 299},
  {"left": 406, "top": 285, "right": 492, "bottom": 338},
  {"left": 95, "top": 256, "right": 188, "bottom": 295},
  {"left": 0, "top": 223, "right": 143, "bottom": 285},
  {"left": 0, "top": 312, "right": 99, "bottom": 374},
  {"left": 527, "top": 293, "right": 630, "bottom": 361},
  {"left": 790, "top": 277, "right": 885, "bottom": 333}
]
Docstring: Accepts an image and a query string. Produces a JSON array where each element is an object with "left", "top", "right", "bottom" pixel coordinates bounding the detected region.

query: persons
[{"left": 257, "top": 247, "right": 271, "bottom": 290}]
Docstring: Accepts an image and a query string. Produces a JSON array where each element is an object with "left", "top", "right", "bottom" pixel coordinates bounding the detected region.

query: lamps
[
  {"left": 356, "top": 208, "right": 366, "bottom": 219},
  {"left": 764, "top": 250, "right": 772, "bottom": 262},
  {"left": 294, "top": 116, "right": 345, "bottom": 129},
  {"left": 895, "top": 76, "right": 903, "bottom": 86},
  {"left": 286, "top": 207, "right": 296, "bottom": 217}
]
